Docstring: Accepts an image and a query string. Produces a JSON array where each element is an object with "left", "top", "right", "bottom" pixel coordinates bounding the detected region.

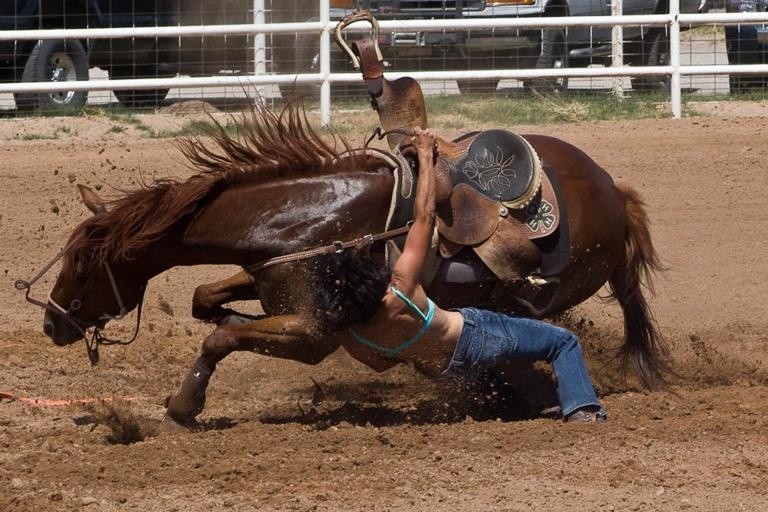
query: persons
[{"left": 314, "top": 125, "right": 606, "bottom": 424}]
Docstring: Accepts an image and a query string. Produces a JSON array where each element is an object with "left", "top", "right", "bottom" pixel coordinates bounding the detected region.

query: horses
[{"left": 40, "top": 79, "right": 684, "bottom": 438}]
